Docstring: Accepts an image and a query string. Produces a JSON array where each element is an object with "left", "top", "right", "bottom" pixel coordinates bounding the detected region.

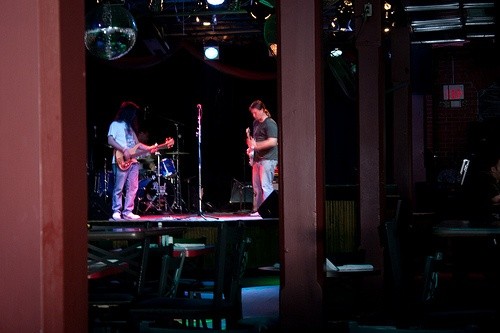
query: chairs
[{"left": 88, "top": 180, "right": 500, "bottom": 333}]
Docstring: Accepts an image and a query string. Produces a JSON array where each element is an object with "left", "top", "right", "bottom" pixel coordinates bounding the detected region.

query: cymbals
[{"left": 165, "top": 152, "right": 190, "bottom": 155}]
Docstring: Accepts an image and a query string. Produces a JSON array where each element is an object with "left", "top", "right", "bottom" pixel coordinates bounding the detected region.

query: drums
[
  {"left": 136, "top": 178, "right": 176, "bottom": 214},
  {"left": 154, "top": 158, "right": 177, "bottom": 178},
  {"left": 97, "top": 170, "right": 116, "bottom": 198}
]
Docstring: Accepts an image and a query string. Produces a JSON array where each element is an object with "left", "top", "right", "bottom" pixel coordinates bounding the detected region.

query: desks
[
  {"left": 88, "top": 225, "right": 186, "bottom": 333},
  {"left": 419, "top": 211, "right": 500, "bottom": 333},
  {"left": 111, "top": 241, "right": 210, "bottom": 333}
]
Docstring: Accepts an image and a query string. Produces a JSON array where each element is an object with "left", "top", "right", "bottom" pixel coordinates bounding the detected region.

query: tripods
[{"left": 142, "top": 125, "right": 219, "bottom": 222}]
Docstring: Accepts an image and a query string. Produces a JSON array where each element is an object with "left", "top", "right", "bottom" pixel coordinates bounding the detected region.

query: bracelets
[{"left": 122, "top": 150, "right": 126, "bottom": 153}]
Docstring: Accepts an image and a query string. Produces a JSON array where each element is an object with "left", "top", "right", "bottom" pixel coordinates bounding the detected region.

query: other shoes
[
  {"left": 123, "top": 211, "right": 141, "bottom": 221},
  {"left": 113, "top": 212, "right": 120, "bottom": 220},
  {"left": 248, "top": 211, "right": 260, "bottom": 217}
]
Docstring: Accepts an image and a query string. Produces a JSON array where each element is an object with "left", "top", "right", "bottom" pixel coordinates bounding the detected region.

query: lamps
[{"left": 203, "top": 39, "right": 220, "bottom": 60}]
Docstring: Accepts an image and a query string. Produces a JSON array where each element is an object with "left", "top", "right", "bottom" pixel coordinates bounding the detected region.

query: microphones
[
  {"left": 196, "top": 104, "right": 201, "bottom": 109},
  {"left": 94, "top": 125, "right": 96, "bottom": 137}
]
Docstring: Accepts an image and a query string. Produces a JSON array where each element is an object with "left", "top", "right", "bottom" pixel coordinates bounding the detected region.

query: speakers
[{"left": 229, "top": 181, "right": 253, "bottom": 211}]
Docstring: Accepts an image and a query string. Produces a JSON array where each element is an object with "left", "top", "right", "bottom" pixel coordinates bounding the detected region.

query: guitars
[
  {"left": 114, "top": 136, "right": 174, "bottom": 172},
  {"left": 246, "top": 127, "right": 256, "bottom": 166}
]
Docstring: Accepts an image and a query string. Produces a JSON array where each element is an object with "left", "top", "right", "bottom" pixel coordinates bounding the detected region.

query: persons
[
  {"left": 245, "top": 100, "right": 279, "bottom": 216},
  {"left": 133, "top": 131, "right": 165, "bottom": 207},
  {"left": 107, "top": 101, "right": 159, "bottom": 220},
  {"left": 417, "top": 143, "right": 490, "bottom": 267},
  {"left": 484, "top": 153, "right": 500, "bottom": 227}
]
